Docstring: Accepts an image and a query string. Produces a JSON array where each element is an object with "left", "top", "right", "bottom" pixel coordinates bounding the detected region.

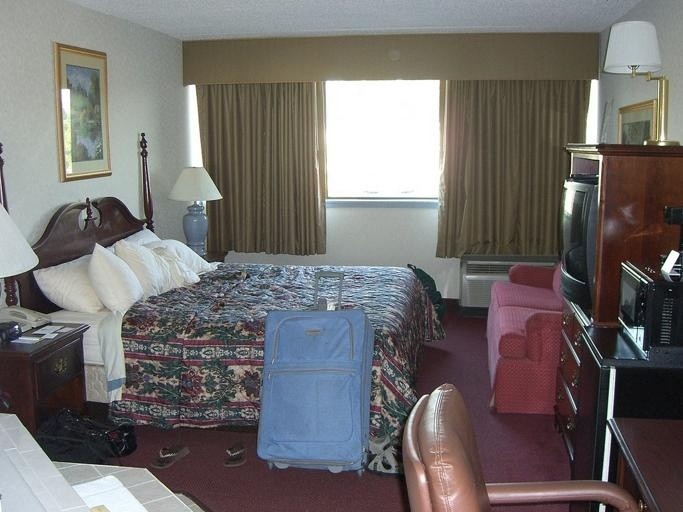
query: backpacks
[{"left": 407, "top": 264, "right": 445, "bottom": 320}]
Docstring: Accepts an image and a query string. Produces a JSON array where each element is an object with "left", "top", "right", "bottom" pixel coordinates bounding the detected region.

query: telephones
[{"left": 0, "top": 305, "right": 52, "bottom": 328}]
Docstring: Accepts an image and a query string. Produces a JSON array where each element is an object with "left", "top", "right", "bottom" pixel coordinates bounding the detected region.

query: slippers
[
  {"left": 222, "top": 443, "right": 246, "bottom": 468},
  {"left": 150, "top": 446, "right": 189, "bottom": 469}
]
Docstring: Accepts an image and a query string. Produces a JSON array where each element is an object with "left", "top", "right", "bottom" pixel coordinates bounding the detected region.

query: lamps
[
  {"left": 0, "top": 205, "right": 41, "bottom": 280},
  {"left": 166, "top": 166, "right": 223, "bottom": 257},
  {"left": 602, "top": 19, "right": 678, "bottom": 145}
]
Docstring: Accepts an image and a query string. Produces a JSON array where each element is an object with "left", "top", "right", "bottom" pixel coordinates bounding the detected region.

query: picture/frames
[
  {"left": 52, "top": 41, "right": 111, "bottom": 183},
  {"left": 615, "top": 99, "right": 659, "bottom": 144}
]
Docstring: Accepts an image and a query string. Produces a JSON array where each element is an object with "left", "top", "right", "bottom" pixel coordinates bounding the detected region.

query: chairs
[{"left": 399, "top": 380, "right": 638, "bottom": 512}]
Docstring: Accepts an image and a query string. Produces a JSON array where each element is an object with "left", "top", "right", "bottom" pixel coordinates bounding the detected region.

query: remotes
[{"left": 571, "top": 174, "right": 596, "bottom": 179}]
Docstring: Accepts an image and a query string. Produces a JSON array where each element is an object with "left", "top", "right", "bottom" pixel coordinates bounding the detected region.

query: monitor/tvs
[{"left": 559, "top": 179, "right": 598, "bottom": 309}]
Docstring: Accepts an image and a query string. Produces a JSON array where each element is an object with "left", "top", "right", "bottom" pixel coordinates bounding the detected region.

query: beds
[{"left": 0, "top": 131, "right": 442, "bottom": 479}]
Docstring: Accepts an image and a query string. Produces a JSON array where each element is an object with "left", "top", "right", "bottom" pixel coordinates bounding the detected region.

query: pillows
[
  {"left": 89, "top": 243, "right": 143, "bottom": 315},
  {"left": 32, "top": 254, "right": 113, "bottom": 314},
  {"left": 106, "top": 228, "right": 164, "bottom": 255},
  {"left": 115, "top": 238, "right": 199, "bottom": 295},
  {"left": 140, "top": 238, "right": 214, "bottom": 275}
]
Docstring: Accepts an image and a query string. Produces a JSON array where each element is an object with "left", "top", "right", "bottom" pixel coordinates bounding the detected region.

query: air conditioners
[{"left": 456, "top": 254, "right": 562, "bottom": 319}]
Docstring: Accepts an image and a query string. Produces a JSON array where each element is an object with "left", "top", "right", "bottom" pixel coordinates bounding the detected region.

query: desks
[{"left": 604, "top": 417, "right": 682, "bottom": 512}]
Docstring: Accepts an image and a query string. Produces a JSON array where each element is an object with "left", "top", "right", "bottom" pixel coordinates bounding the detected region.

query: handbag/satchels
[{"left": 32, "top": 408, "right": 137, "bottom": 458}]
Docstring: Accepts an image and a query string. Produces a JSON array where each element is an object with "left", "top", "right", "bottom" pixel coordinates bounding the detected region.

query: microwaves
[{"left": 615, "top": 260, "right": 682, "bottom": 363}]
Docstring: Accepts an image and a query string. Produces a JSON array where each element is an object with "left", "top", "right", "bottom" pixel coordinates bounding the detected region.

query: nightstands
[
  {"left": 200, "top": 250, "right": 227, "bottom": 264},
  {"left": 0, "top": 321, "right": 90, "bottom": 438}
]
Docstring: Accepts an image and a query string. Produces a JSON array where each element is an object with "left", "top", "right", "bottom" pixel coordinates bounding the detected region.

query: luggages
[{"left": 256, "top": 272, "right": 373, "bottom": 477}]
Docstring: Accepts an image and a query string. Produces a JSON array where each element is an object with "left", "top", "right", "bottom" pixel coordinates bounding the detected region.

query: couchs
[{"left": 484, "top": 262, "right": 563, "bottom": 417}]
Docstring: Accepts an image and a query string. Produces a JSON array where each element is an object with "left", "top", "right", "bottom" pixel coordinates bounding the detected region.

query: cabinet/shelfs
[
  {"left": 554, "top": 295, "right": 682, "bottom": 512},
  {"left": 561, "top": 139, "right": 682, "bottom": 329}
]
